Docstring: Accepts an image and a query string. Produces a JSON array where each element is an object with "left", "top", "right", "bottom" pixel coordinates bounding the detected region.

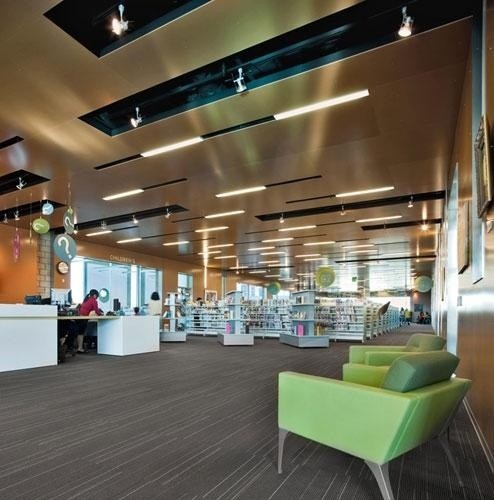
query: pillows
[
  {"left": 403, "top": 333, "right": 446, "bottom": 351},
  {"left": 380, "top": 350, "right": 461, "bottom": 393}
]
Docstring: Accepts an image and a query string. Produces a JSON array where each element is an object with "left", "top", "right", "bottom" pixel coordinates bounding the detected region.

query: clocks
[{"left": 57, "top": 261, "right": 69, "bottom": 274}]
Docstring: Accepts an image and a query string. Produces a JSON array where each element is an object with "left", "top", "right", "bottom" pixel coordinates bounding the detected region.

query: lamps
[
  {"left": 130, "top": 7, "right": 414, "bottom": 128},
  {"left": 2, "top": 210, "right": 20, "bottom": 224},
  {"left": 16, "top": 178, "right": 24, "bottom": 190},
  {"left": 111, "top": 5, "right": 129, "bottom": 36},
  {"left": 279, "top": 193, "right": 414, "bottom": 224},
  {"left": 74, "top": 208, "right": 170, "bottom": 234}
]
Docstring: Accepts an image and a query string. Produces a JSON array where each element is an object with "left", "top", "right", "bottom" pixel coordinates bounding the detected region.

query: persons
[
  {"left": 400, "top": 307, "right": 430, "bottom": 326},
  {"left": 73, "top": 289, "right": 103, "bottom": 353},
  {"left": 148, "top": 292, "right": 162, "bottom": 315},
  {"left": 134, "top": 308, "right": 139, "bottom": 315}
]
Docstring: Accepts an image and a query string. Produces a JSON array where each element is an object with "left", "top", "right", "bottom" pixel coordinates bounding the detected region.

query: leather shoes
[{"left": 76, "top": 350, "right": 89, "bottom": 354}]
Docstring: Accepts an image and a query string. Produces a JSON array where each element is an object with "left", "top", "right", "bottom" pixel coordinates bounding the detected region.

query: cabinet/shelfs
[{"left": 158, "top": 290, "right": 399, "bottom": 347}]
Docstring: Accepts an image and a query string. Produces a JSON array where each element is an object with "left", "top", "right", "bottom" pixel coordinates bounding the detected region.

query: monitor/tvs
[
  {"left": 26, "top": 295, "right": 42, "bottom": 305},
  {"left": 50, "top": 288, "right": 72, "bottom": 312},
  {"left": 113, "top": 299, "right": 120, "bottom": 311}
]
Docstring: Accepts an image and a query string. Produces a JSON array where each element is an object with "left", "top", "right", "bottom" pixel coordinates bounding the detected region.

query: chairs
[
  {"left": 277, "top": 352, "right": 472, "bottom": 500},
  {"left": 349, "top": 333, "right": 446, "bottom": 365}
]
